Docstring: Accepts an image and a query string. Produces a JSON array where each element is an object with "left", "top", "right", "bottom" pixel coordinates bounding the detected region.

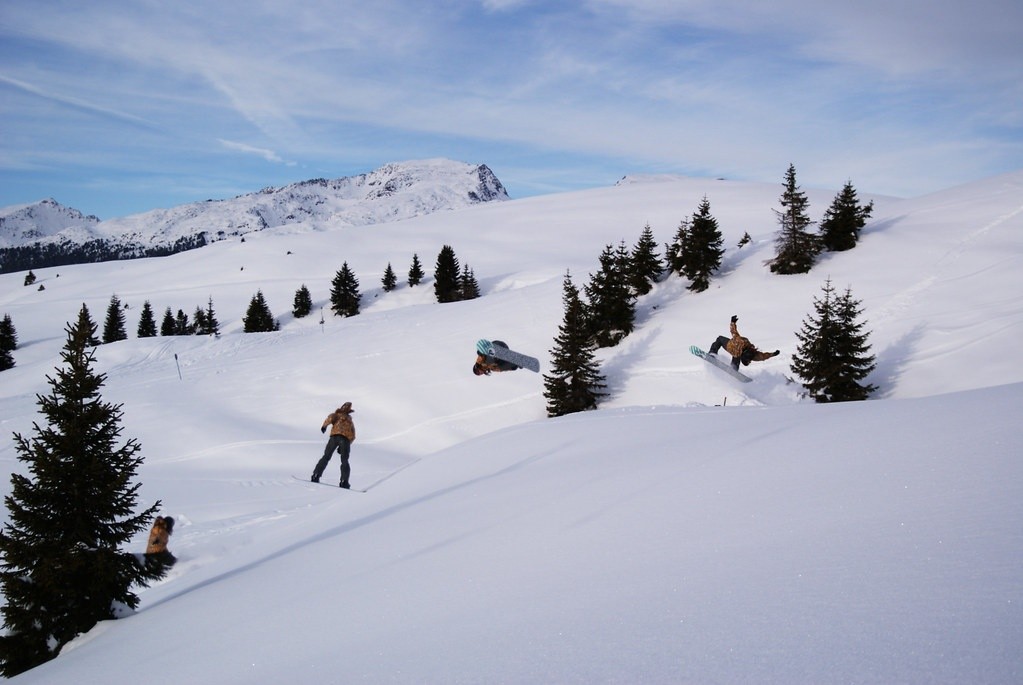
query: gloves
[
  {"left": 731, "top": 315, "right": 738, "bottom": 323},
  {"left": 337, "top": 447, "right": 341, "bottom": 454},
  {"left": 152, "top": 540, "right": 159, "bottom": 545},
  {"left": 774, "top": 350, "right": 780, "bottom": 356},
  {"left": 320, "top": 426, "right": 326, "bottom": 433}
]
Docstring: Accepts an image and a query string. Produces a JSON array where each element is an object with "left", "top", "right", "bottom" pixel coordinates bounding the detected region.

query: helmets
[{"left": 473, "top": 365, "right": 479, "bottom": 375}]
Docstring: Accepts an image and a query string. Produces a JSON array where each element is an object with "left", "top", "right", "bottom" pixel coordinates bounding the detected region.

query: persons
[
  {"left": 145, "top": 516, "right": 177, "bottom": 566},
  {"left": 311, "top": 401, "right": 356, "bottom": 489},
  {"left": 708, "top": 314, "right": 780, "bottom": 372},
  {"left": 472, "top": 340, "right": 522, "bottom": 376}
]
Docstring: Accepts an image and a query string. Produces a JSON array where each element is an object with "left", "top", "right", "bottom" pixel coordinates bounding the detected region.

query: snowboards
[
  {"left": 690, "top": 344, "right": 754, "bottom": 383},
  {"left": 291, "top": 472, "right": 367, "bottom": 493},
  {"left": 476, "top": 339, "right": 540, "bottom": 373}
]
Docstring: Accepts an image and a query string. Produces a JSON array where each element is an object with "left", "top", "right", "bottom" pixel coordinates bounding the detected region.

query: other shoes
[
  {"left": 311, "top": 476, "right": 319, "bottom": 483},
  {"left": 340, "top": 482, "right": 350, "bottom": 488},
  {"left": 708, "top": 352, "right": 717, "bottom": 357}
]
